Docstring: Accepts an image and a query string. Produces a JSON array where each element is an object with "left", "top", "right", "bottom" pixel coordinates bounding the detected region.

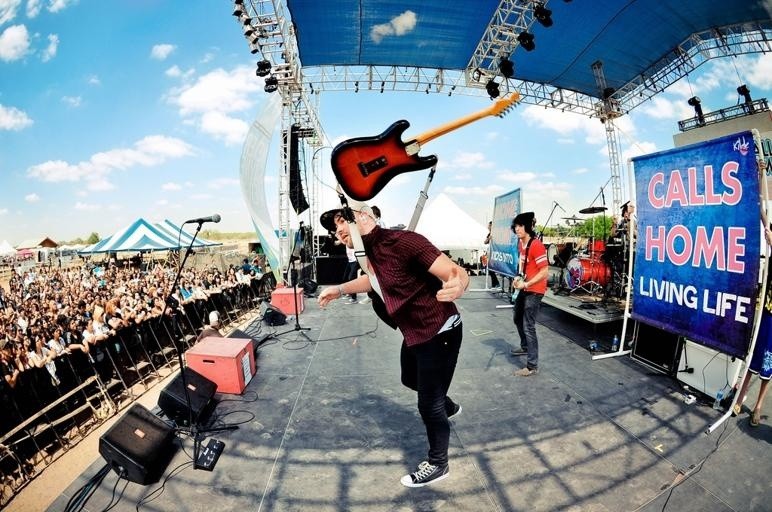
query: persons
[
  {"left": 507, "top": 211, "right": 548, "bottom": 376},
  {"left": 313, "top": 199, "right": 469, "bottom": 489},
  {"left": 1, "top": 258, "right": 273, "bottom": 484},
  {"left": 484, "top": 220, "right": 501, "bottom": 289}
]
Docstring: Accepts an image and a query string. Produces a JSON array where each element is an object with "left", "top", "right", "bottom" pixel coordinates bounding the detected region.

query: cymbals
[{"left": 579, "top": 207, "right": 608, "bottom": 213}]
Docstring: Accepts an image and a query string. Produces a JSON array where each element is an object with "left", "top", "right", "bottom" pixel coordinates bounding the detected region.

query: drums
[
  {"left": 547, "top": 243, "right": 566, "bottom": 266},
  {"left": 565, "top": 258, "right": 610, "bottom": 289}
]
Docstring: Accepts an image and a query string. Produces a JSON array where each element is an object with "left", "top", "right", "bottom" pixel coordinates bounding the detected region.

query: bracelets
[
  {"left": 338, "top": 285, "right": 344, "bottom": 296},
  {"left": 523, "top": 282, "right": 527, "bottom": 288}
]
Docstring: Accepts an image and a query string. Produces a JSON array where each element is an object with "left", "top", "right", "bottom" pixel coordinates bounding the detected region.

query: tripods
[
  {"left": 274, "top": 262, "right": 312, "bottom": 343},
  {"left": 165, "top": 301, "right": 240, "bottom": 472},
  {"left": 567, "top": 221, "right": 603, "bottom": 296}
]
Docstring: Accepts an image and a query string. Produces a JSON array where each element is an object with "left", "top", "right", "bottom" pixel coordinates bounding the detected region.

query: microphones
[
  {"left": 186, "top": 213, "right": 223, "bottom": 228},
  {"left": 553, "top": 199, "right": 567, "bottom": 214},
  {"left": 600, "top": 186, "right": 606, "bottom": 205}
]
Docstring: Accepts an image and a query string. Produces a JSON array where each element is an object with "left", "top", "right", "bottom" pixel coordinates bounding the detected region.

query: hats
[
  {"left": 0, "top": 339, "right": 10, "bottom": 350},
  {"left": 209, "top": 310, "right": 220, "bottom": 327},
  {"left": 319, "top": 202, "right": 377, "bottom": 228}
]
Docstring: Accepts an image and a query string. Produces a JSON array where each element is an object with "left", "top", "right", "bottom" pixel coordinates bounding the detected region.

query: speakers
[
  {"left": 259, "top": 300, "right": 287, "bottom": 325},
  {"left": 98, "top": 401, "right": 176, "bottom": 486},
  {"left": 156, "top": 365, "right": 219, "bottom": 427},
  {"left": 227, "top": 329, "right": 259, "bottom": 352},
  {"left": 303, "top": 278, "right": 319, "bottom": 293}
]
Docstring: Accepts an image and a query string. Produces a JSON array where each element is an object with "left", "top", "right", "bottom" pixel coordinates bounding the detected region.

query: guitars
[
  {"left": 331, "top": 91, "right": 521, "bottom": 201},
  {"left": 511, "top": 273, "right": 527, "bottom": 302}
]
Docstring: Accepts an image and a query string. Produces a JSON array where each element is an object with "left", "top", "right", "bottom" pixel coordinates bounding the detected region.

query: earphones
[{"left": 360, "top": 215, "right": 367, "bottom": 223}]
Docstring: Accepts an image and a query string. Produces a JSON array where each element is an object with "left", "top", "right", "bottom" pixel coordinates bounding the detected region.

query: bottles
[
  {"left": 713, "top": 389, "right": 724, "bottom": 410},
  {"left": 612, "top": 335, "right": 618, "bottom": 351}
]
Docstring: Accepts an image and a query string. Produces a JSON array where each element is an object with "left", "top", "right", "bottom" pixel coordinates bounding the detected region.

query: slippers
[
  {"left": 751, "top": 410, "right": 760, "bottom": 426},
  {"left": 732, "top": 399, "right": 742, "bottom": 416}
]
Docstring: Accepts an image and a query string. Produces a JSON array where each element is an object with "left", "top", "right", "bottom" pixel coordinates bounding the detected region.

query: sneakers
[
  {"left": 341, "top": 295, "right": 352, "bottom": 300},
  {"left": 515, "top": 367, "right": 538, "bottom": 376},
  {"left": 509, "top": 346, "right": 528, "bottom": 355},
  {"left": 345, "top": 298, "right": 358, "bottom": 305},
  {"left": 400, "top": 460, "right": 450, "bottom": 488},
  {"left": 446, "top": 399, "right": 462, "bottom": 420}
]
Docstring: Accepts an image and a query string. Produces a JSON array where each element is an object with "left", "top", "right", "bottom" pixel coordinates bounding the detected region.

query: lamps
[
  {"left": 255, "top": 60, "right": 278, "bottom": 94},
  {"left": 485, "top": 2, "right": 553, "bottom": 100}
]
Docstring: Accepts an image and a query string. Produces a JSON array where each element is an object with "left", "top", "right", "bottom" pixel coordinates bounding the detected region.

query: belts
[{"left": 445, "top": 316, "right": 462, "bottom": 330}]
